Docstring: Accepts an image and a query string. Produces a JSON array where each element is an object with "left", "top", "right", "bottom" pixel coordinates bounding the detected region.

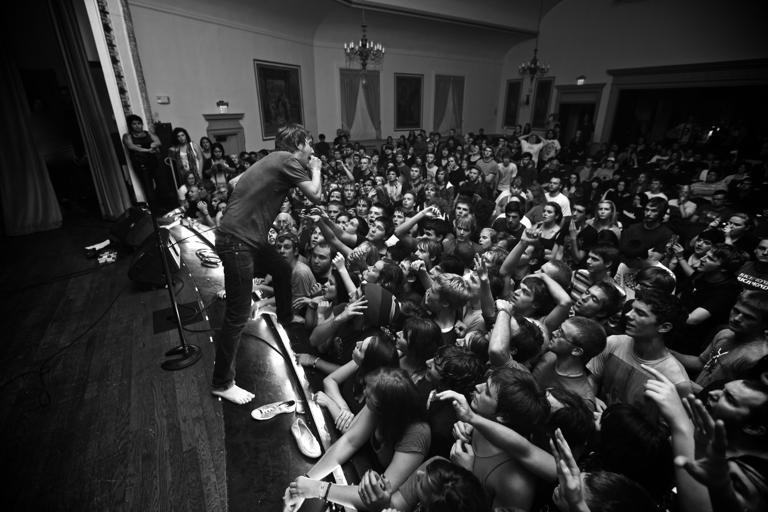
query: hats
[{"left": 364, "top": 281, "right": 403, "bottom": 327}]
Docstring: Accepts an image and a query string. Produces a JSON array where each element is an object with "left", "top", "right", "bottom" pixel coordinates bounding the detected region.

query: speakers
[
  {"left": 143, "top": 228, "right": 180, "bottom": 273},
  {"left": 127, "top": 242, "right": 172, "bottom": 290},
  {"left": 110, "top": 207, "right": 155, "bottom": 248}
]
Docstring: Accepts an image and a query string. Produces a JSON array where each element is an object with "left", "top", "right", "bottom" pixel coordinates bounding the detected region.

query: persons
[
  {"left": 179, "top": 108, "right": 768, "bottom": 511},
  {"left": 121, "top": 114, "right": 179, "bottom": 215},
  {"left": 205, "top": 122, "right": 324, "bottom": 406},
  {"left": 165, "top": 127, "right": 206, "bottom": 187}
]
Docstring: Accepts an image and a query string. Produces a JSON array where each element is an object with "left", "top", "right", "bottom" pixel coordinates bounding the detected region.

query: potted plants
[{"left": 216, "top": 100, "right": 228, "bottom": 113}]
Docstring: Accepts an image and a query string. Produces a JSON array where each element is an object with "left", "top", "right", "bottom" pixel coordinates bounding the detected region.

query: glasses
[
  {"left": 634, "top": 280, "right": 658, "bottom": 291},
  {"left": 556, "top": 324, "right": 580, "bottom": 348}
]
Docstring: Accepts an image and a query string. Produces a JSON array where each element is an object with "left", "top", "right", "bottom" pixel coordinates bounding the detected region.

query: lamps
[
  {"left": 576, "top": 75, "right": 585, "bottom": 88},
  {"left": 516, "top": 0, "right": 551, "bottom": 84},
  {"left": 344, "top": 8, "right": 385, "bottom": 89}
]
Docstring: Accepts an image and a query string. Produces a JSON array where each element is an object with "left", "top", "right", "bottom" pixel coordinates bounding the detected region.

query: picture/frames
[
  {"left": 253, "top": 59, "right": 305, "bottom": 141},
  {"left": 530, "top": 77, "right": 555, "bottom": 131},
  {"left": 394, "top": 72, "right": 424, "bottom": 131},
  {"left": 502, "top": 79, "right": 522, "bottom": 129}
]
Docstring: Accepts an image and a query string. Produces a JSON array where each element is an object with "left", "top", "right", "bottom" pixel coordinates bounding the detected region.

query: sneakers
[
  {"left": 290, "top": 417, "right": 321, "bottom": 459},
  {"left": 250, "top": 398, "right": 299, "bottom": 421}
]
